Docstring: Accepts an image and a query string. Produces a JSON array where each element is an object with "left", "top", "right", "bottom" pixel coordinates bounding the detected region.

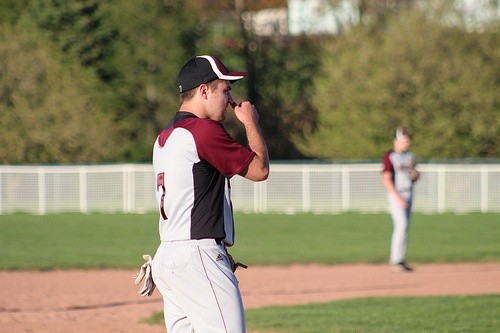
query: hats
[
  {"left": 394, "top": 128, "right": 412, "bottom": 138},
  {"left": 179, "top": 54, "right": 246, "bottom": 93}
]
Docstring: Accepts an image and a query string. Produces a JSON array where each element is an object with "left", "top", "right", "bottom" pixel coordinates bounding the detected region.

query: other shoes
[{"left": 391, "top": 260, "right": 414, "bottom": 273}]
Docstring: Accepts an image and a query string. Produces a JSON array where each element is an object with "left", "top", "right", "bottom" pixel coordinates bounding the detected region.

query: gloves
[{"left": 133, "top": 253, "right": 158, "bottom": 298}]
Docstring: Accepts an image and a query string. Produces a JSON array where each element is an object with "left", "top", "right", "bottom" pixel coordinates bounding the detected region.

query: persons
[
  {"left": 381, "top": 127, "right": 420, "bottom": 271},
  {"left": 151, "top": 54, "right": 270, "bottom": 333}
]
